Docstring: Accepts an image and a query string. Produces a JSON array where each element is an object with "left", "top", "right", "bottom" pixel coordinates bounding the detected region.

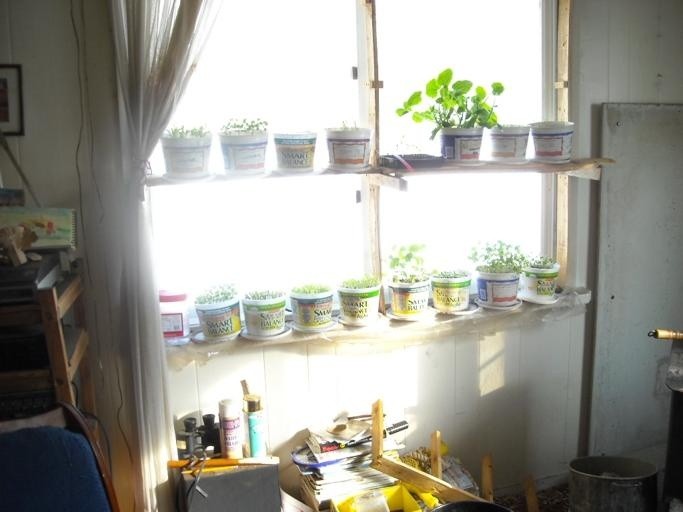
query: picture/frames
[{"left": 0, "top": 64, "right": 24, "bottom": 135}]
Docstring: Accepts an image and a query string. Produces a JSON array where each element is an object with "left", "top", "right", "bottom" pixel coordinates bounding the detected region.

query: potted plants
[
  {"left": 396, "top": 68, "right": 505, "bottom": 165},
  {"left": 386, "top": 243, "right": 433, "bottom": 318},
  {"left": 218, "top": 116, "right": 269, "bottom": 177},
  {"left": 158, "top": 126, "right": 213, "bottom": 182},
  {"left": 289, "top": 282, "right": 334, "bottom": 332},
  {"left": 336, "top": 275, "right": 382, "bottom": 323},
  {"left": 242, "top": 289, "right": 288, "bottom": 337},
  {"left": 519, "top": 254, "right": 560, "bottom": 303},
  {"left": 468, "top": 239, "right": 529, "bottom": 307},
  {"left": 432, "top": 269, "right": 471, "bottom": 313},
  {"left": 193, "top": 283, "right": 241, "bottom": 339}
]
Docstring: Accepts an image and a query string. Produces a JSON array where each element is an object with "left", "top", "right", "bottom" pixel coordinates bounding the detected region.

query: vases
[
  {"left": 491, "top": 122, "right": 530, "bottom": 165},
  {"left": 325, "top": 119, "right": 373, "bottom": 172},
  {"left": 275, "top": 131, "right": 316, "bottom": 174},
  {"left": 530, "top": 120, "right": 575, "bottom": 165}
]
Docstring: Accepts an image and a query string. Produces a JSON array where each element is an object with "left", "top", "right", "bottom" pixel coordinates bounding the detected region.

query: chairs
[{"left": 0, "top": 398, "right": 121, "bottom": 512}]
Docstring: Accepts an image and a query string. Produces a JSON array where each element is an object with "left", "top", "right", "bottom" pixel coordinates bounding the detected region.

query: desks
[{"left": 659, "top": 336, "right": 683, "bottom": 512}]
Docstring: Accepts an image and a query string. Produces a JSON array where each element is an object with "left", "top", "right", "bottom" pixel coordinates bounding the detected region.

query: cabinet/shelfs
[
  {"left": 140, "top": 155, "right": 614, "bottom": 367},
  {"left": 0, "top": 275, "right": 120, "bottom": 512}
]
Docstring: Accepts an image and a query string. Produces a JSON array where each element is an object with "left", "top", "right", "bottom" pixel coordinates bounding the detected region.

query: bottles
[
  {"left": 183, "top": 395, "right": 263, "bottom": 459},
  {"left": 158, "top": 290, "right": 188, "bottom": 339}
]
